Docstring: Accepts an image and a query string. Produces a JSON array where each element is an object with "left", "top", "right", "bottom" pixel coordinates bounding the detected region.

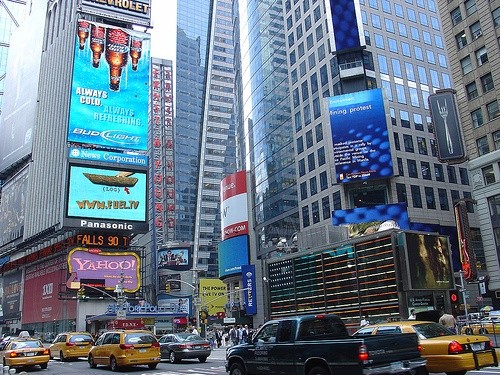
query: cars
[
  {"left": 466, "top": 313, "right": 484, "bottom": 323},
  {"left": 0, "top": 338, "right": 50, "bottom": 370},
  {"left": 351, "top": 320, "right": 500, "bottom": 375},
  {"left": 468, "top": 312, "right": 484, "bottom": 321},
  {"left": 0, "top": 335, "right": 19, "bottom": 350},
  {"left": 481, "top": 309, "right": 500, "bottom": 319},
  {"left": 44, "top": 333, "right": 55, "bottom": 342},
  {"left": 157, "top": 333, "right": 211, "bottom": 364},
  {"left": 456, "top": 315, "right": 475, "bottom": 324},
  {"left": 460, "top": 320, "right": 500, "bottom": 335},
  {"left": 31, "top": 333, "right": 43, "bottom": 342}
]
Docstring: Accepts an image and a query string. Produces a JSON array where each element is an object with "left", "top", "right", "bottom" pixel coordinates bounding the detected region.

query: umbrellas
[{"left": 480, "top": 305, "right": 494, "bottom": 311}]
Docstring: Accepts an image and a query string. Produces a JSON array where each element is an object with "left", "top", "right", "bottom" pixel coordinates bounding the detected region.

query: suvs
[
  {"left": 48, "top": 332, "right": 94, "bottom": 361},
  {"left": 87, "top": 330, "right": 161, "bottom": 371}
]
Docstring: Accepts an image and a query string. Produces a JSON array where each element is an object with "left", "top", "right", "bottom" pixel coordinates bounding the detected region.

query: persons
[
  {"left": 407, "top": 309, "right": 417, "bottom": 321},
  {"left": 439, "top": 307, "right": 458, "bottom": 336},
  {"left": 361, "top": 315, "right": 369, "bottom": 328},
  {"left": 170, "top": 322, "right": 265, "bottom": 350},
  {"left": 158, "top": 250, "right": 188, "bottom": 266}
]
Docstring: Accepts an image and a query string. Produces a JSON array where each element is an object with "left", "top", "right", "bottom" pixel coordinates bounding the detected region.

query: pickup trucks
[{"left": 224, "top": 314, "right": 428, "bottom": 375}]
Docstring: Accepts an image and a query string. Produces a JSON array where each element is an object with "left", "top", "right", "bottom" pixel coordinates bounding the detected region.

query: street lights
[{"left": 167, "top": 278, "right": 200, "bottom": 335}]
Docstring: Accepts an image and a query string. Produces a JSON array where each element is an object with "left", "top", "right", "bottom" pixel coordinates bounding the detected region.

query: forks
[{"left": 437, "top": 98, "right": 453, "bottom": 154}]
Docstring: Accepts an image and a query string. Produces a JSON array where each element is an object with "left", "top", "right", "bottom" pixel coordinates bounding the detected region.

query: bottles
[
  {"left": 77, "top": 21, "right": 90, "bottom": 50},
  {"left": 130, "top": 36, "right": 142, "bottom": 70},
  {"left": 105, "top": 28, "right": 130, "bottom": 90},
  {"left": 90, "top": 23, "right": 105, "bottom": 68}
]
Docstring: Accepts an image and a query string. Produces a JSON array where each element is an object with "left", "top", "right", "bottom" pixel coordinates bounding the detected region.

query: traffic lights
[{"left": 449, "top": 290, "right": 460, "bottom": 310}]
